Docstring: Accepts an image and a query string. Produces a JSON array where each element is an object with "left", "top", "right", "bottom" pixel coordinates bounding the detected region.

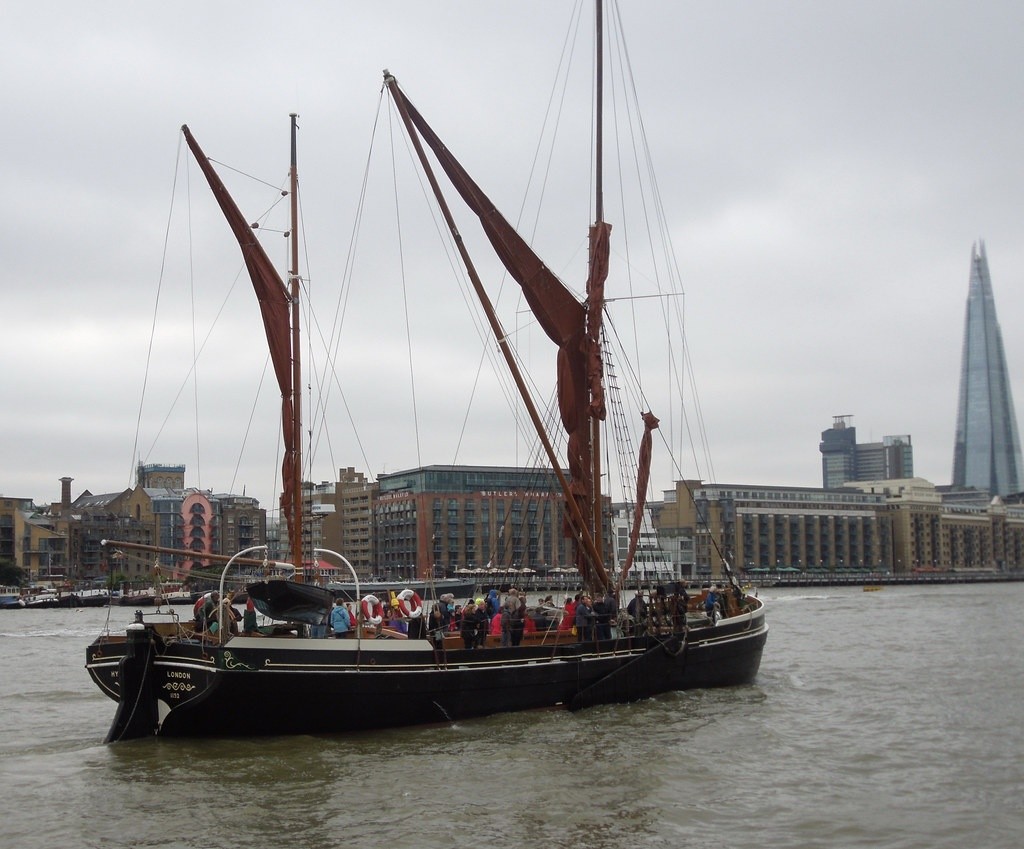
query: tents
[{"left": 452, "top": 567, "right": 609, "bottom": 582}]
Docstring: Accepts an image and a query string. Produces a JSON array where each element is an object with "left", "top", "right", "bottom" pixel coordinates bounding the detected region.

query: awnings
[{"left": 747, "top": 566, "right": 879, "bottom": 572}]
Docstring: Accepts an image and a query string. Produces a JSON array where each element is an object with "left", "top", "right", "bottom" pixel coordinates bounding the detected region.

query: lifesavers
[
  {"left": 361, "top": 594, "right": 384, "bottom": 625},
  {"left": 397, "top": 587, "right": 424, "bottom": 618}
]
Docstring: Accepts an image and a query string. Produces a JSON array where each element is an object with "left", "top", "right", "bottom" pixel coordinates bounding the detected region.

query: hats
[{"left": 545, "top": 596, "right": 552, "bottom": 602}]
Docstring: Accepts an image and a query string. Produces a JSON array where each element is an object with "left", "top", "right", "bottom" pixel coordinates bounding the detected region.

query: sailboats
[{"left": 86, "top": 0, "right": 771, "bottom": 743}]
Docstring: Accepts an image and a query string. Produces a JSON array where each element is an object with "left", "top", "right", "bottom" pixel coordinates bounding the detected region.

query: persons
[
  {"left": 329, "top": 579, "right": 718, "bottom": 649},
  {"left": 198, "top": 589, "right": 242, "bottom": 636}
]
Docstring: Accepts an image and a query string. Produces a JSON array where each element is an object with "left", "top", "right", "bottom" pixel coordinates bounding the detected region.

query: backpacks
[{"left": 485, "top": 597, "right": 496, "bottom": 616}]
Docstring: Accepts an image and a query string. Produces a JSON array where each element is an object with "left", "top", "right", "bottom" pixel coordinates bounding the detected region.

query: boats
[{"left": 0, "top": 586, "right": 195, "bottom": 610}]
